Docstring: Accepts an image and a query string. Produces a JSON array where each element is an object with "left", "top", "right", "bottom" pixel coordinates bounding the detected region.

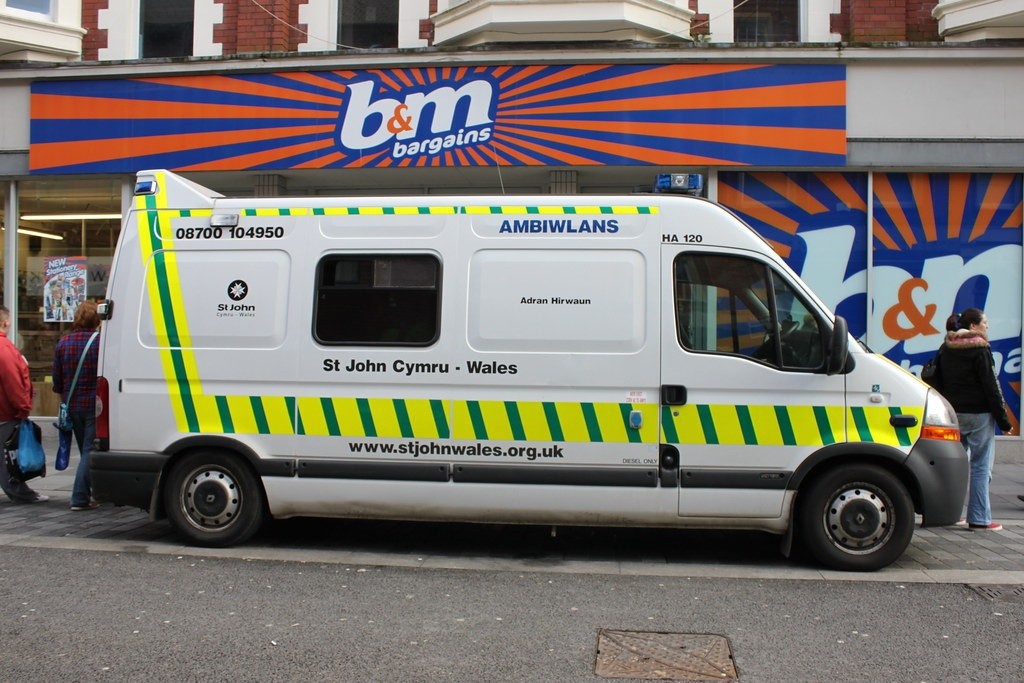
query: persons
[
  {"left": 0, "top": 306, "right": 50, "bottom": 503},
  {"left": 920, "top": 308, "right": 1014, "bottom": 531},
  {"left": 800, "top": 314, "right": 820, "bottom": 334},
  {"left": 52, "top": 300, "right": 101, "bottom": 509}
]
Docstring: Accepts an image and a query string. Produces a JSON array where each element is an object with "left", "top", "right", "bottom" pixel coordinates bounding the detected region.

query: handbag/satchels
[
  {"left": 58, "top": 402, "right": 69, "bottom": 429},
  {"left": 4, "top": 418, "right": 42, "bottom": 484},
  {"left": 52, "top": 421, "right": 72, "bottom": 470},
  {"left": 920, "top": 342, "right": 947, "bottom": 385},
  {"left": 18, "top": 420, "right": 46, "bottom": 481}
]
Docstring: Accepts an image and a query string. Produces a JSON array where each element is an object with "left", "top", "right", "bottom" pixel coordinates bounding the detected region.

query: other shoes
[
  {"left": 969, "top": 522, "right": 1003, "bottom": 532},
  {"left": 71, "top": 502, "right": 100, "bottom": 510},
  {"left": 30, "top": 492, "right": 50, "bottom": 504}
]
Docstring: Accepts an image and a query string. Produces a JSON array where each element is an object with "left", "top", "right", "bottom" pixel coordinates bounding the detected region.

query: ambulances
[{"left": 89, "top": 169, "right": 970, "bottom": 572}]
